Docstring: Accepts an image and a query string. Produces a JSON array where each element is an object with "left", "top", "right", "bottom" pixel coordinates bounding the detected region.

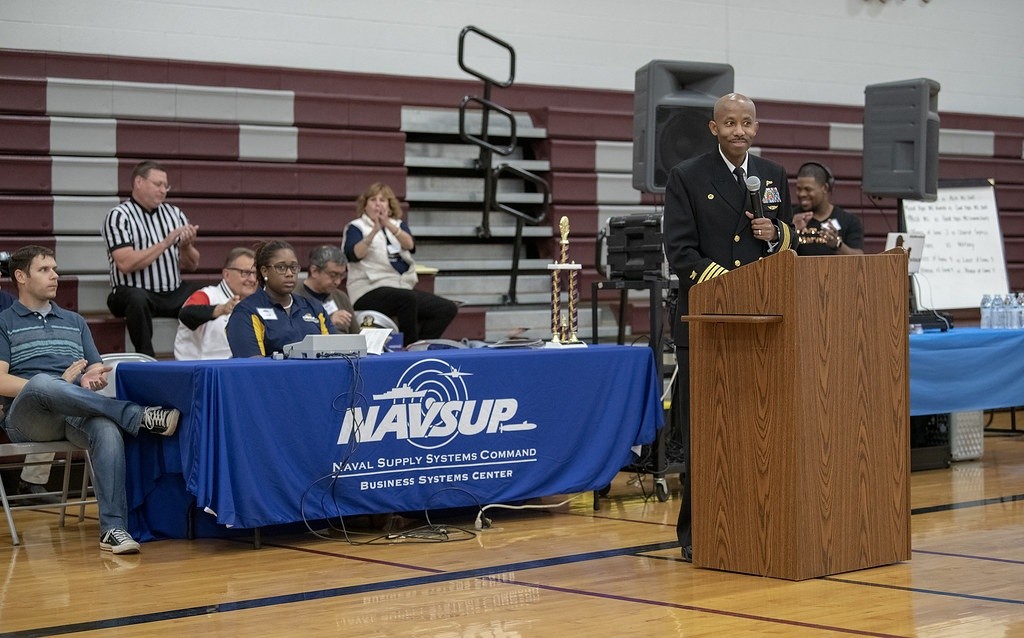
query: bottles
[{"left": 980, "top": 293, "right": 1024, "bottom": 329}]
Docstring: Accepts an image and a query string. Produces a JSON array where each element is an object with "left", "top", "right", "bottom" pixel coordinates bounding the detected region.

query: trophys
[{"left": 545, "top": 216, "right": 589, "bottom": 348}]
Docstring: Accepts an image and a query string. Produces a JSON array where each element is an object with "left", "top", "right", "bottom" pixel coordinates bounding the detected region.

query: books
[{"left": 352, "top": 327, "right": 394, "bottom": 355}]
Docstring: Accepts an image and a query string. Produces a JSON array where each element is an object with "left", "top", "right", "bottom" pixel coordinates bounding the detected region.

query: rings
[{"left": 758, "top": 230, "right": 762, "bottom": 236}]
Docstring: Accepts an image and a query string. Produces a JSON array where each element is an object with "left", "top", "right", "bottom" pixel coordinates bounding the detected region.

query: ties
[{"left": 732, "top": 166, "right": 750, "bottom": 195}]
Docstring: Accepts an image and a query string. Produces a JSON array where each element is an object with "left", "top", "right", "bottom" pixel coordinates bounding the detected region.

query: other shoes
[
  {"left": 16, "top": 480, "right": 63, "bottom": 504},
  {"left": 680, "top": 544, "right": 693, "bottom": 561}
]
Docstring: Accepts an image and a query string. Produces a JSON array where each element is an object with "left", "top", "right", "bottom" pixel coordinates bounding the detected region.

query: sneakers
[
  {"left": 99, "top": 527, "right": 142, "bottom": 553},
  {"left": 137, "top": 406, "right": 181, "bottom": 435}
]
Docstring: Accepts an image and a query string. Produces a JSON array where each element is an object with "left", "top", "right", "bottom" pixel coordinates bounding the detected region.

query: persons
[
  {"left": 340, "top": 182, "right": 458, "bottom": 348},
  {"left": 292, "top": 247, "right": 361, "bottom": 336},
  {"left": 0, "top": 246, "right": 180, "bottom": 554},
  {"left": 174, "top": 247, "right": 258, "bottom": 360},
  {"left": 101, "top": 162, "right": 200, "bottom": 358},
  {"left": 225, "top": 239, "right": 338, "bottom": 359},
  {"left": 664, "top": 93, "right": 800, "bottom": 562},
  {"left": 788, "top": 161, "right": 864, "bottom": 258}
]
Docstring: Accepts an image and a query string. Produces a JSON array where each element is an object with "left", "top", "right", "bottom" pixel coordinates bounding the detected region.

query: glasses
[
  {"left": 315, "top": 264, "right": 348, "bottom": 282},
  {"left": 226, "top": 266, "right": 260, "bottom": 280},
  {"left": 137, "top": 170, "right": 172, "bottom": 195},
  {"left": 264, "top": 263, "right": 302, "bottom": 275}
]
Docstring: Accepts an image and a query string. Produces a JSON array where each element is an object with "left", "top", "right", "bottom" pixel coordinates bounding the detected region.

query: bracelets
[
  {"left": 393, "top": 228, "right": 400, "bottom": 237},
  {"left": 768, "top": 224, "right": 781, "bottom": 249},
  {"left": 362, "top": 238, "right": 372, "bottom": 246}
]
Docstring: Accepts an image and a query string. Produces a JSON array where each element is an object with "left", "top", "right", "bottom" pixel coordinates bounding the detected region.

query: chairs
[
  {"left": 78, "top": 352, "right": 158, "bottom": 524},
  {"left": 0, "top": 441, "right": 101, "bottom": 546}
]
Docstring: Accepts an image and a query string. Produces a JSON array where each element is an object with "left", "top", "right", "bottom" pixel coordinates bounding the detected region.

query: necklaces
[{"left": 283, "top": 293, "right": 294, "bottom": 311}]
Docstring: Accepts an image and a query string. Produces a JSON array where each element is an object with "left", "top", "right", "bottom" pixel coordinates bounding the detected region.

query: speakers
[
  {"left": 861, "top": 77, "right": 941, "bottom": 202},
  {"left": 632, "top": 60, "right": 735, "bottom": 195}
]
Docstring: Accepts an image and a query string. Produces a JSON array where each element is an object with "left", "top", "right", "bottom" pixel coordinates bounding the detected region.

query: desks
[
  {"left": 115, "top": 342, "right": 665, "bottom": 549},
  {"left": 908, "top": 326, "right": 1024, "bottom": 433}
]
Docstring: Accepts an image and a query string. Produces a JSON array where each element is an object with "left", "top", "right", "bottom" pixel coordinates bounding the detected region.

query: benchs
[{"left": 0, "top": 46, "right": 1023, "bottom": 358}]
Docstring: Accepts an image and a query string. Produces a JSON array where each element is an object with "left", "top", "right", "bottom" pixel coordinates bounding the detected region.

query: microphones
[{"left": 746, "top": 176, "right": 763, "bottom": 219}]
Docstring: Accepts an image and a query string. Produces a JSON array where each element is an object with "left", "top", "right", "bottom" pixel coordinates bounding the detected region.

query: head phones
[{"left": 797, "top": 160, "right": 835, "bottom": 193}]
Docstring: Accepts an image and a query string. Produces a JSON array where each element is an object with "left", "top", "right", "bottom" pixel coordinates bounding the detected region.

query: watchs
[{"left": 829, "top": 237, "right": 843, "bottom": 251}]
